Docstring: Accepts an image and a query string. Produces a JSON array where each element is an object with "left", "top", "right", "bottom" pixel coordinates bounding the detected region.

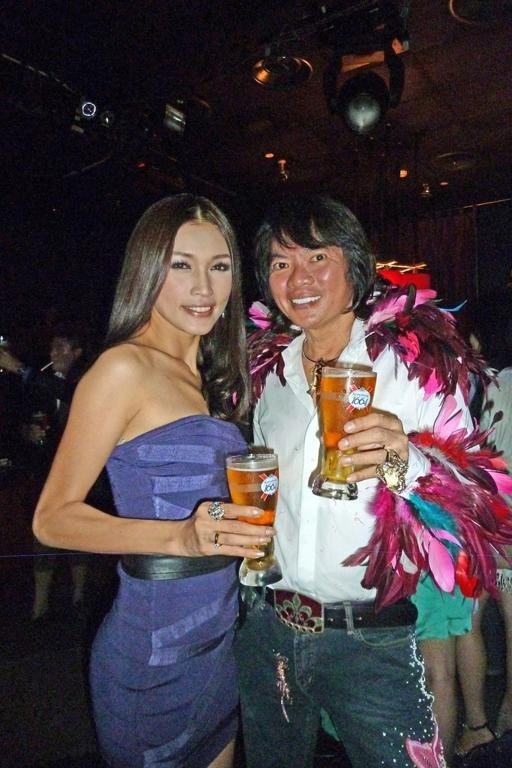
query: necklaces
[{"left": 300, "top": 334, "right": 341, "bottom": 395}]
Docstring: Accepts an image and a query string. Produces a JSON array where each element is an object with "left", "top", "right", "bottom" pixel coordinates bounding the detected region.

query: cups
[
  {"left": 312, "top": 366, "right": 377, "bottom": 501},
  {"left": 225, "top": 454, "right": 283, "bottom": 586},
  {"left": 31, "top": 409, "right": 49, "bottom": 448}
]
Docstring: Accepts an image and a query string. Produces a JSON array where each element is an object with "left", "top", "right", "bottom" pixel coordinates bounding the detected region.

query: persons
[
  {"left": 237, "top": 198, "right": 481, "bottom": 767},
  {"left": 451, "top": 317, "right": 511, "bottom": 768},
  {"left": 29, "top": 190, "right": 275, "bottom": 768},
  {"left": 409, "top": 562, "right": 476, "bottom": 767},
  {"left": 1, "top": 328, "right": 89, "bottom": 478},
  {"left": 22, "top": 477, "right": 96, "bottom": 626}
]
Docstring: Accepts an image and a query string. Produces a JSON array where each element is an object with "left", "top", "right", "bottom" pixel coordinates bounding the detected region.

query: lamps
[
  {"left": 78, "top": 96, "right": 116, "bottom": 130},
  {"left": 323, "top": 34, "right": 405, "bottom": 138}
]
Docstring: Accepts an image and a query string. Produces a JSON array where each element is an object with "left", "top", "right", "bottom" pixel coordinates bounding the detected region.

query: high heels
[{"left": 453, "top": 724, "right": 497, "bottom": 767}]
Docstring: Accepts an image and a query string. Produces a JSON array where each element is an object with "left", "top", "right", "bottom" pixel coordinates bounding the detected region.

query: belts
[{"left": 259, "top": 589, "right": 418, "bottom": 634}]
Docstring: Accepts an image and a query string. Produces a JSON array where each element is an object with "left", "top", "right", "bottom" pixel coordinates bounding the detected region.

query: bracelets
[{"left": 15, "top": 362, "right": 30, "bottom": 378}]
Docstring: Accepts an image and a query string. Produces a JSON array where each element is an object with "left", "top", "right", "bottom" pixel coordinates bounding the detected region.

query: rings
[
  {"left": 207, "top": 500, "right": 230, "bottom": 521},
  {"left": 375, "top": 447, "right": 407, "bottom": 494},
  {"left": 212, "top": 533, "right": 221, "bottom": 544}
]
